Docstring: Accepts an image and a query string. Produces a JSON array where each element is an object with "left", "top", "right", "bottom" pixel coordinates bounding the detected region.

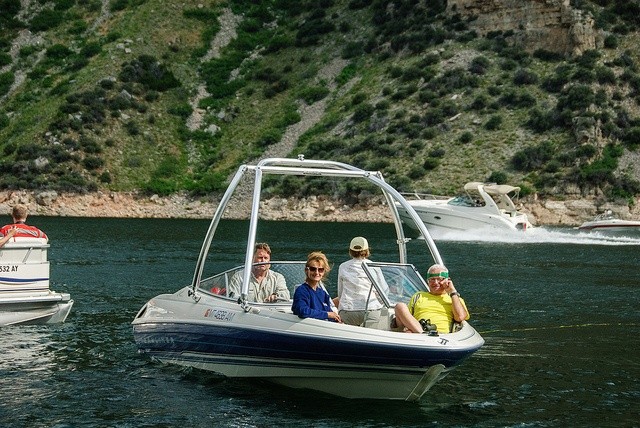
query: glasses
[
  {"left": 427, "top": 278, "right": 445, "bottom": 284},
  {"left": 306, "top": 265, "right": 326, "bottom": 274}
]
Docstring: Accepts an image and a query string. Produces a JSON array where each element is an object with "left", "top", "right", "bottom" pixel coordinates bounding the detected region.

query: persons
[
  {"left": 394, "top": 264, "right": 470, "bottom": 335},
  {"left": 0, "top": 225, "right": 19, "bottom": 249},
  {"left": 292, "top": 252, "right": 341, "bottom": 323},
  {"left": 230, "top": 243, "right": 290, "bottom": 303},
  {"left": 0, "top": 205, "right": 49, "bottom": 242},
  {"left": 337, "top": 237, "right": 388, "bottom": 329}
]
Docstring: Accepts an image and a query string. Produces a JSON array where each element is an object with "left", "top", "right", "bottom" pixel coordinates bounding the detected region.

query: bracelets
[{"left": 449, "top": 291, "right": 459, "bottom": 297}]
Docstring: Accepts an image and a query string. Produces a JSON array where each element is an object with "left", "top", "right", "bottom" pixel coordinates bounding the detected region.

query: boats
[
  {"left": 1, "top": 236, "right": 74, "bottom": 330},
  {"left": 130, "top": 154, "right": 487, "bottom": 402},
  {"left": 394, "top": 181, "right": 534, "bottom": 239},
  {"left": 579, "top": 211, "right": 640, "bottom": 232}
]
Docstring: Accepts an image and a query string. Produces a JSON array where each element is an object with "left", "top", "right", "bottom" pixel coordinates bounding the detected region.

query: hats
[{"left": 349, "top": 236, "right": 369, "bottom": 251}]
